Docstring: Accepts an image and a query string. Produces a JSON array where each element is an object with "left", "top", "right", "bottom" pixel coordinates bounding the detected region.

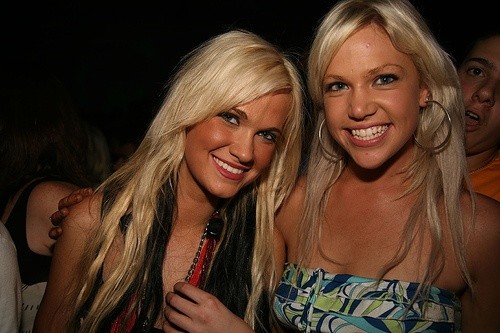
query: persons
[
  {"left": 48, "top": 1, "right": 500, "bottom": 333},
  {"left": 458, "top": 33, "right": 499, "bottom": 200},
  {"left": 31, "top": 30, "right": 306, "bottom": 332},
  {"left": 1, "top": 93, "right": 146, "bottom": 333}
]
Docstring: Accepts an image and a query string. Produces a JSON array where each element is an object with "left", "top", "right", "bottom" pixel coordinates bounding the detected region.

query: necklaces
[{"left": 184, "top": 213, "right": 213, "bottom": 283}]
